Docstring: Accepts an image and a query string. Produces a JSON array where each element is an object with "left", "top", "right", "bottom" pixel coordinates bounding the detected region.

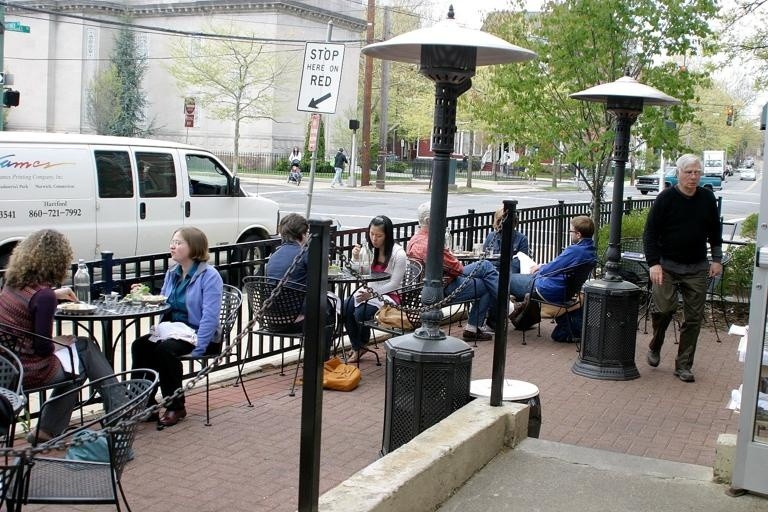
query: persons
[
  {"left": 1, "top": 229, "right": 138, "bottom": 443},
  {"left": 288, "top": 166, "right": 303, "bottom": 186},
  {"left": 481, "top": 216, "right": 595, "bottom": 332},
  {"left": 289, "top": 146, "right": 302, "bottom": 163},
  {"left": 130, "top": 226, "right": 222, "bottom": 425},
  {"left": 264, "top": 213, "right": 332, "bottom": 360},
  {"left": 642, "top": 153, "right": 722, "bottom": 382},
  {"left": 340, "top": 215, "right": 406, "bottom": 362},
  {"left": 459, "top": 153, "right": 468, "bottom": 173},
  {"left": 506, "top": 156, "right": 512, "bottom": 175},
  {"left": 330, "top": 148, "right": 350, "bottom": 188},
  {"left": 482, "top": 207, "right": 528, "bottom": 275},
  {"left": 406, "top": 203, "right": 499, "bottom": 341}
]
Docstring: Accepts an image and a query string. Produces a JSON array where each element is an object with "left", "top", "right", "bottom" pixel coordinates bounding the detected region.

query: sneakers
[
  {"left": 673, "top": 367, "right": 695, "bottom": 383},
  {"left": 646, "top": 346, "right": 660, "bottom": 367}
]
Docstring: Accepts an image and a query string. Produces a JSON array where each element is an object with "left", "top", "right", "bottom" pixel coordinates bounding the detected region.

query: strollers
[{"left": 288, "top": 158, "right": 304, "bottom": 186}]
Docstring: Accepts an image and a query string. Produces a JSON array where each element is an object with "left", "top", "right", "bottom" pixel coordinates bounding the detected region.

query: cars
[
  {"left": 726, "top": 164, "right": 733, "bottom": 176},
  {"left": 739, "top": 169, "right": 756, "bottom": 181},
  {"left": 736, "top": 156, "right": 755, "bottom": 172}
]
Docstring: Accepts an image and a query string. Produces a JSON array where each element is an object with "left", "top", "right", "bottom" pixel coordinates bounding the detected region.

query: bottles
[
  {"left": 444, "top": 227, "right": 453, "bottom": 253},
  {"left": 73, "top": 258, "right": 91, "bottom": 305},
  {"left": 358, "top": 241, "right": 372, "bottom": 276}
]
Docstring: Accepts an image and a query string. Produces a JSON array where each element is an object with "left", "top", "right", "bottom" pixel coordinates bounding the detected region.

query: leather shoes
[
  {"left": 160, "top": 408, "right": 187, "bottom": 426},
  {"left": 462, "top": 330, "right": 492, "bottom": 341},
  {"left": 27, "top": 433, "right": 49, "bottom": 444},
  {"left": 347, "top": 343, "right": 369, "bottom": 363}
]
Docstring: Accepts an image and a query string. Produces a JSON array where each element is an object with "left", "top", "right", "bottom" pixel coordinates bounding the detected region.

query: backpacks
[{"left": 296, "top": 356, "right": 364, "bottom": 390}]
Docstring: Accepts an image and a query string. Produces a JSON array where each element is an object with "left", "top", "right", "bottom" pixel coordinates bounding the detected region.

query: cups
[
  {"left": 131, "top": 291, "right": 142, "bottom": 307},
  {"left": 104, "top": 295, "right": 116, "bottom": 311},
  {"left": 454, "top": 242, "right": 493, "bottom": 256}
]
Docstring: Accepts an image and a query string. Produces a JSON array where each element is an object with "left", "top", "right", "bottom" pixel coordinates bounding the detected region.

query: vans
[{"left": 0, "top": 131, "right": 280, "bottom": 286}]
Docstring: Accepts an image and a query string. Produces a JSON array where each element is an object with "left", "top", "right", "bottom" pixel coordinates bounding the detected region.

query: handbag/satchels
[
  {"left": 550, "top": 306, "right": 583, "bottom": 343},
  {"left": 375, "top": 301, "right": 421, "bottom": 330},
  {"left": 508, "top": 300, "right": 542, "bottom": 331},
  {"left": 63, "top": 427, "right": 135, "bottom": 470}
]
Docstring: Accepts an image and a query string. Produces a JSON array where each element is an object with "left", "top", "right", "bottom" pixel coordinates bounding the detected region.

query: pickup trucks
[{"left": 636, "top": 167, "right": 721, "bottom": 196}]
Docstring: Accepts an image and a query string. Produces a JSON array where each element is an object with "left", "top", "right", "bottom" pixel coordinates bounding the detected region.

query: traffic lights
[
  {"left": 349, "top": 120, "right": 359, "bottom": 129},
  {"left": 733, "top": 109, "right": 740, "bottom": 122},
  {"left": 726, "top": 105, "right": 734, "bottom": 127},
  {"left": 3, "top": 89, "right": 20, "bottom": 107}
]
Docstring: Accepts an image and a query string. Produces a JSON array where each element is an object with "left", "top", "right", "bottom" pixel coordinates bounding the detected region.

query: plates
[
  {"left": 132, "top": 296, "right": 167, "bottom": 304},
  {"left": 57, "top": 307, "right": 99, "bottom": 314}
]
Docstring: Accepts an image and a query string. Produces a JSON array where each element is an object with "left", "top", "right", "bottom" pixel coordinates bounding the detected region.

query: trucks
[{"left": 703, "top": 150, "right": 726, "bottom": 180}]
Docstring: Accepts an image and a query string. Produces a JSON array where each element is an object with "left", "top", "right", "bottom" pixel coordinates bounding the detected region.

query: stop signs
[{"left": 185, "top": 98, "right": 196, "bottom": 115}]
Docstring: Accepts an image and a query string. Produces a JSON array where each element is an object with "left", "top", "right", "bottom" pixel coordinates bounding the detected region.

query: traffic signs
[{"left": 5, "top": 21, "right": 30, "bottom": 33}]
